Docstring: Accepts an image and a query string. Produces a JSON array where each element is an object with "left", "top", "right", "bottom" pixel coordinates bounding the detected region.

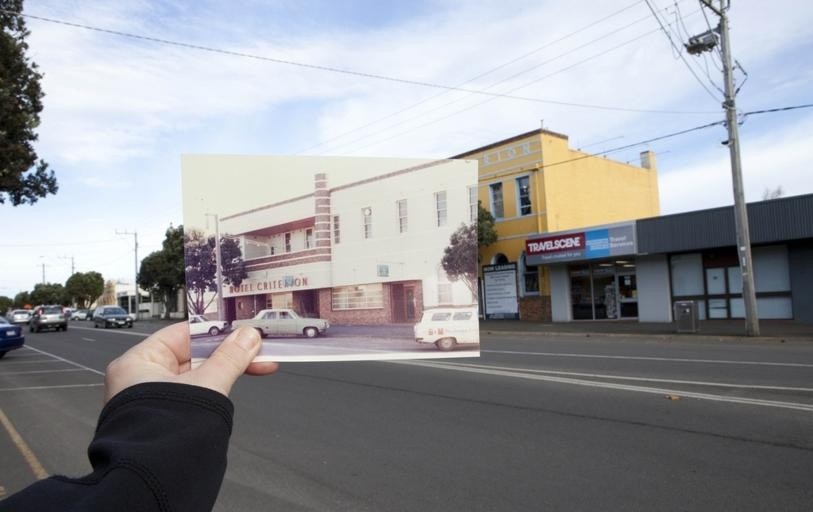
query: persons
[{"left": 0, "top": 319, "right": 281, "bottom": 512}]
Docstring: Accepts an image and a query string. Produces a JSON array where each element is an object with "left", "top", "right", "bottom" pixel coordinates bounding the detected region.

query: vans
[{"left": 414, "top": 304, "right": 479, "bottom": 352}]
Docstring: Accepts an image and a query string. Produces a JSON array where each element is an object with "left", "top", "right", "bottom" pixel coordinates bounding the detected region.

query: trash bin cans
[{"left": 673, "top": 300, "right": 700, "bottom": 334}]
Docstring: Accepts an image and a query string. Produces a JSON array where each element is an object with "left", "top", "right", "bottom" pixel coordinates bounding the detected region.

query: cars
[
  {"left": 0, "top": 304, "right": 134, "bottom": 356},
  {"left": 190, "top": 313, "right": 229, "bottom": 338},
  {"left": 232, "top": 308, "right": 328, "bottom": 340}
]
